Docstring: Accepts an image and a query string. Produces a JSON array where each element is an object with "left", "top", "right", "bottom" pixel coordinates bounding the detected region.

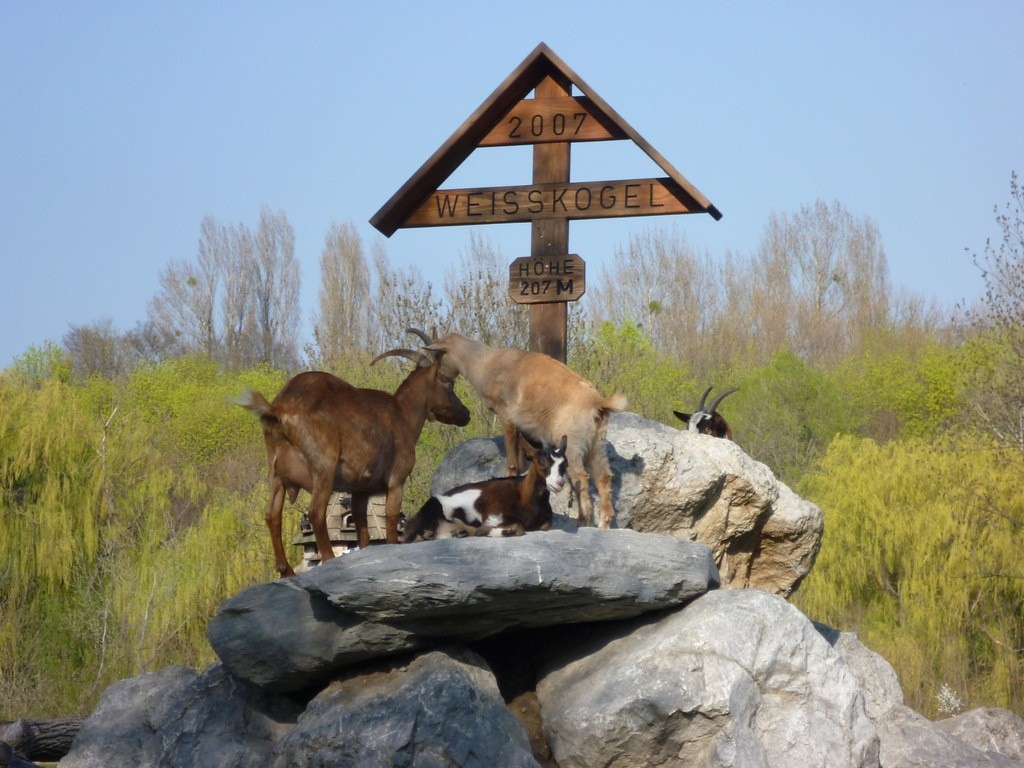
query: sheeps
[
  {"left": 399, "top": 327, "right": 630, "bottom": 544},
  {"left": 234, "top": 345, "right": 472, "bottom": 578},
  {"left": 670, "top": 384, "right": 744, "bottom": 444}
]
